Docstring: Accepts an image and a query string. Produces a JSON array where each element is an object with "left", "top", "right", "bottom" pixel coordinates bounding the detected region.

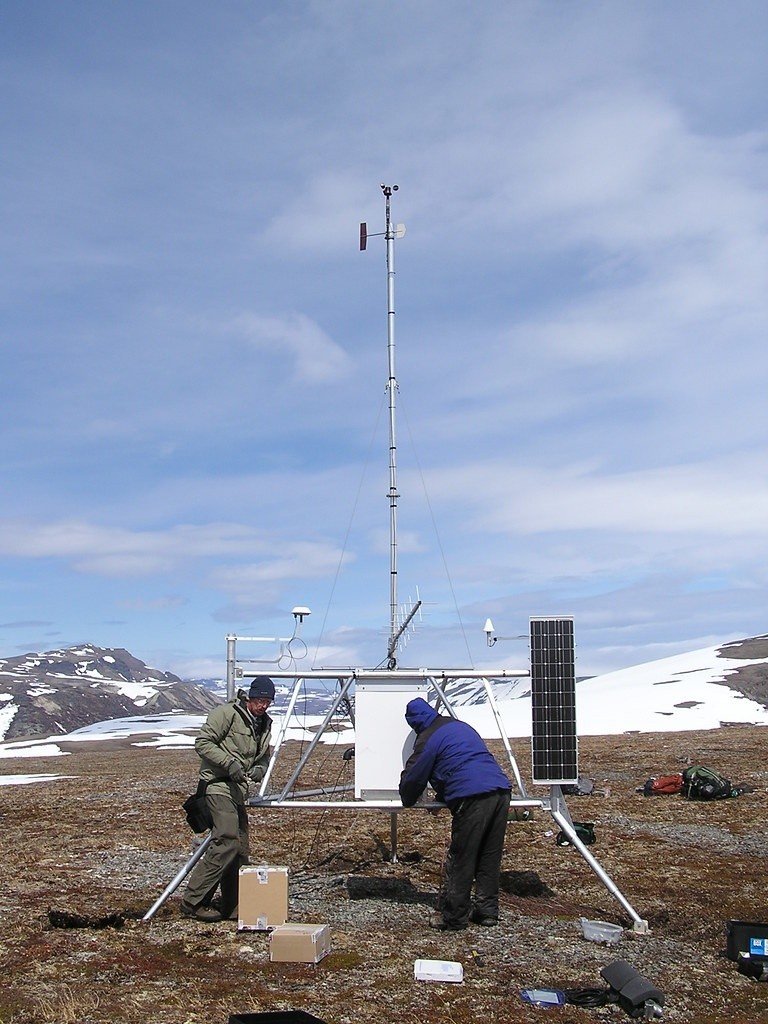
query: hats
[{"left": 249, "top": 676, "right": 276, "bottom": 700}]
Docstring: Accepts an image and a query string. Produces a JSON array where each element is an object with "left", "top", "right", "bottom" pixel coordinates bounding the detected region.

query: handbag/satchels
[
  {"left": 556, "top": 822, "right": 596, "bottom": 847},
  {"left": 181, "top": 779, "right": 220, "bottom": 833}
]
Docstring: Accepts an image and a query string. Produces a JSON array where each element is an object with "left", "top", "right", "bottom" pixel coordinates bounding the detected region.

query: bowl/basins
[{"left": 581, "top": 920, "right": 624, "bottom": 943}]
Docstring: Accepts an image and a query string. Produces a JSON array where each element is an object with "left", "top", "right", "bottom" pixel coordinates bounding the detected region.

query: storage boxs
[
  {"left": 581, "top": 920, "right": 624, "bottom": 944},
  {"left": 238, "top": 865, "right": 290, "bottom": 931},
  {"left": 725, "top": 920, "right": 768, "bottom": 962},
  {"left": 269, "top": 923, "right": 332, "bottom": 964}
]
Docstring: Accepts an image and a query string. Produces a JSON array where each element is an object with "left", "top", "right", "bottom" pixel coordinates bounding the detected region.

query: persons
[
  {"left": 399, "top": 697, "right": 512, "bottom": 929},
  {"left": 179, "top": 676, "right": 276, "bottom": 921}
]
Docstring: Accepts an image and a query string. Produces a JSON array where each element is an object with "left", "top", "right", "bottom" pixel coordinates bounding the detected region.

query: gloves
[
  {"left": 247, "top": 766, "right": 264, "bottom": 783},
  {"left": 229, "top": 759, "right": 245, "bottom": 784}
]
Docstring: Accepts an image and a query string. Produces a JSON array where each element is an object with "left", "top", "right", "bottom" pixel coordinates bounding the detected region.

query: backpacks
[{"left": 681, "top": 764, "right": 732, "bottom": 799}]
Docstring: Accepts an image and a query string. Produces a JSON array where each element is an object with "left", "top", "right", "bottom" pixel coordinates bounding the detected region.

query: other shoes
[
  {"left": 430, "top": 916, "right": 468, "bottom": 930},
  {"left": 469, "top": 910, "right": 497, "bottom": 926}
]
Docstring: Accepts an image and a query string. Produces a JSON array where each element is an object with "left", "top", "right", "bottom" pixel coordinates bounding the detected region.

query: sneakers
[
  {"left": 179, "top": 900, "right": 222, "bottom": 921},
  {"left": 222, "top": 903, "right": 239, "bottom": 920}
]
